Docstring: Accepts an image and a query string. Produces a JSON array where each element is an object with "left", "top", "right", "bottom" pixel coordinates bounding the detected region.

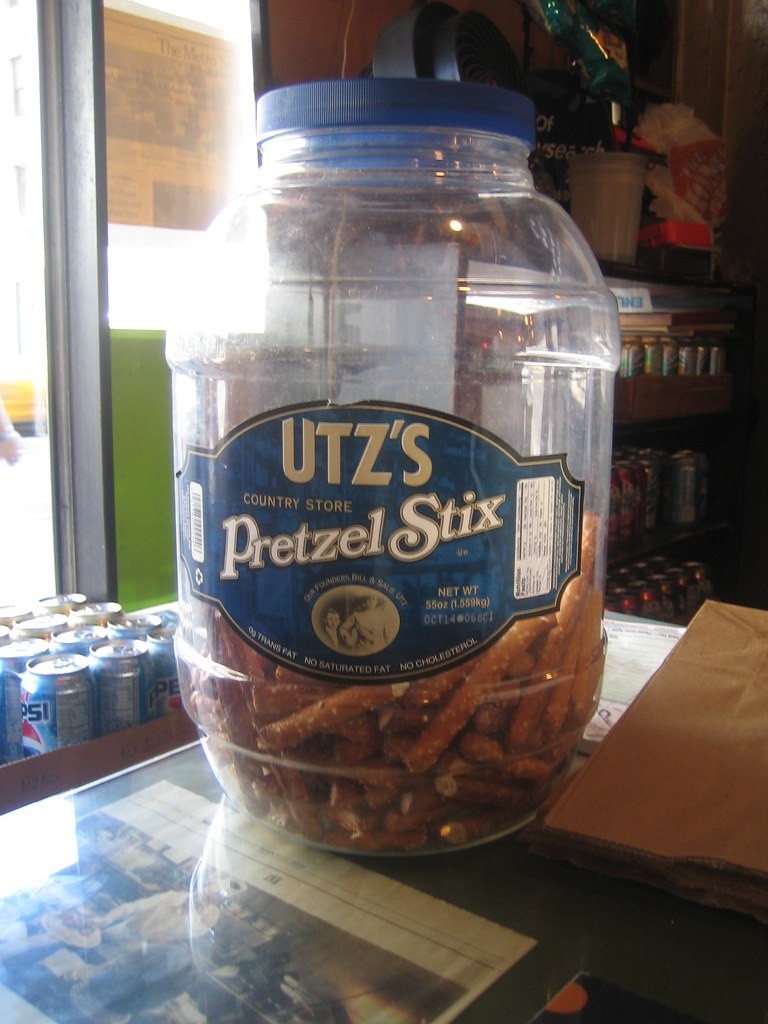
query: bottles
[{"left": 164, "top": 77, "right": 620, "bottom": 857}]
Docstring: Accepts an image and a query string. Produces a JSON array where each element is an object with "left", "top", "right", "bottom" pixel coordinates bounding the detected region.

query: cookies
[{"left": 188, "top": 507, "right": 605, "bottom": 853}]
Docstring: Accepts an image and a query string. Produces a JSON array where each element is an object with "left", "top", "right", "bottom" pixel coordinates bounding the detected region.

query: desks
[{"left": 0, "top": 608, "right": 768, "bottom": 1024}]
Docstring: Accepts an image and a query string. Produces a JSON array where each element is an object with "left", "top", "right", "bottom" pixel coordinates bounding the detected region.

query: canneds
[
  {"left": 0, "top": 592, "right": 181, "bottom": 767},
  {"left": 617, "top": 337, "right": 727, "bottom": 377},
  {"left": 607, "top": 441, "right": 711, "bottom": 625}
]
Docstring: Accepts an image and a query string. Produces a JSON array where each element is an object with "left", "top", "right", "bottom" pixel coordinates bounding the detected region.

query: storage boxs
[{"left": 0, "top": 710, "right": 199, "bottom": 815}]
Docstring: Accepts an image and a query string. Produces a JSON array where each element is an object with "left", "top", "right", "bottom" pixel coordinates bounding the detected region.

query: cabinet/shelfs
[{"left": 245, "top": 0, "right": 768, "bottom": 622}]
